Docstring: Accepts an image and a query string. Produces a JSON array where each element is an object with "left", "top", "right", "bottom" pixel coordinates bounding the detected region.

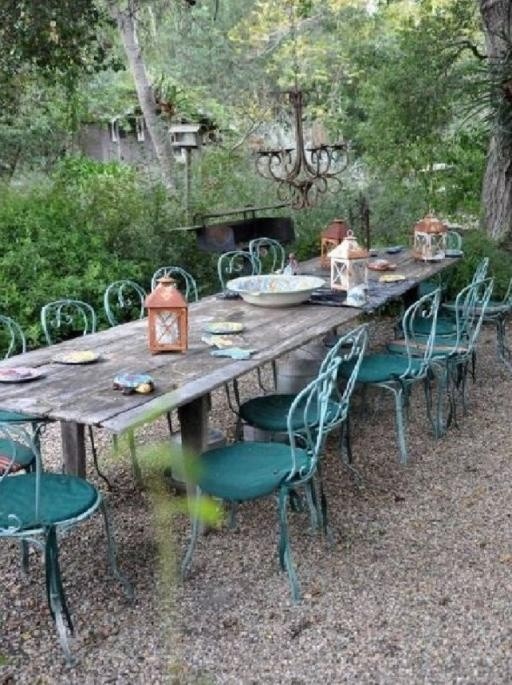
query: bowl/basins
[{"left": 227, "top": 274, "right": 326, "bottom": 307}]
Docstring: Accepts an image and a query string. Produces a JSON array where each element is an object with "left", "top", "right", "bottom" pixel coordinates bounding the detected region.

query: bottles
[{"left": 283, "top": 253, "right": 302, "bottom": 275}]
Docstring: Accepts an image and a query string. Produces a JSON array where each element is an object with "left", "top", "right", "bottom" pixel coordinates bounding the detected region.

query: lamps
[
  {"left": 413, "top": 207, "right": 445, "bottom": 263},
  {"left": 320, "top": 217, "right": 354, "bottom": 271}
]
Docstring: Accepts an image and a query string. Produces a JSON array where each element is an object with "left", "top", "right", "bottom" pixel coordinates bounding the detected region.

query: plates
[
  {"left": 0, "top": 365, "right": 42, "bottom": 383},
  {"left": 50, "top": 349, "right": 101, "bottom": 365},
  {"left": 206, "top": 320, "right": 247, "bottom": 334}
]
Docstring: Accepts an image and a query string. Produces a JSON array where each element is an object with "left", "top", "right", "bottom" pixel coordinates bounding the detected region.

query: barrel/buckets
[
  {"left": 242, "top": 418, "right": 272, "bottom": 443},
  {"left": 169, "top": 423, "right": 227, "bottom": 481},
  {"left": 276, "top": 354, "right": 320, "bottom": 395}
]
